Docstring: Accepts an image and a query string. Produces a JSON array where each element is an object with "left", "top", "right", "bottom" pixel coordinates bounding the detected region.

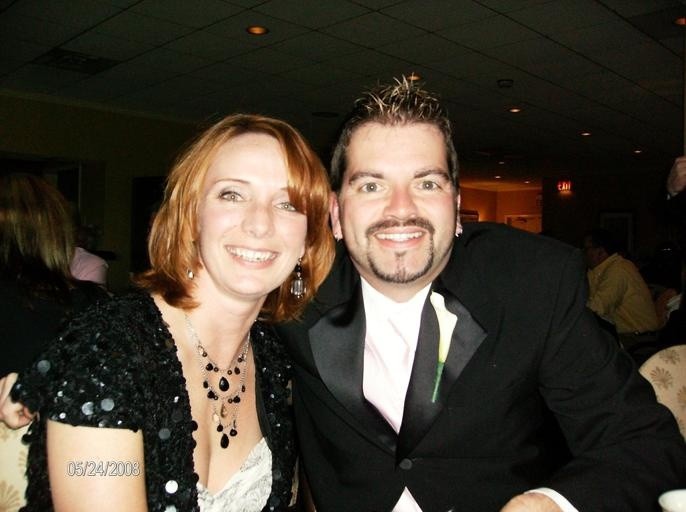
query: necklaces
[{"left": 185, "top": 318, "right": 249, "bottom": 454}]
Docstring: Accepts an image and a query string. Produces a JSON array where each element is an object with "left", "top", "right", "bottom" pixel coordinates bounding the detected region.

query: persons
[
  {"left": 659, "top": 155, "right": 686, "bottom": 346},
  {"left": 575, "top": 225, "right": 664, "bottom": 352},
  {"left": 0, "top": 169, "right": 120, "bottom": 378},
  {"left": 8, "top": 106, "right": 339, "bottom": 510},
  {"left": 63, "top": 197, "right": 115, "bottom": 297},
  {"left": 0, "top": 68, "right": 686, "bottom": 511},
  {"left": 144, "top": 197, "right": 164, "bottom": 248}
]
